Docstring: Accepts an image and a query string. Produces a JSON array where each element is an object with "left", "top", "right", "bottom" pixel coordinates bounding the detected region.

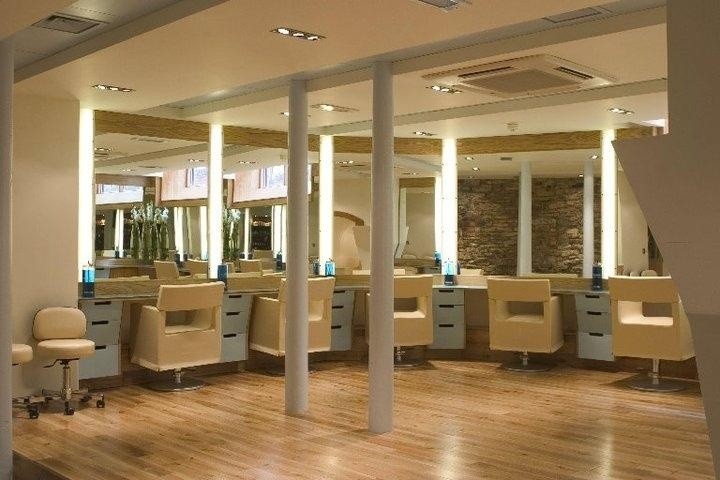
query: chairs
[
  {"left": 226, "top": 249, "right": 277, "bottom": 274},
  {"left": 488, "top": 278, "right": 564, "bottom": 372},
  {"left": 250, "top": 277, "right": 335, "bottom": 375},
  {"left": 364, "top": 275, "right": 433, "bottom": 366},
  {"left": 609, "top": 276, "right": 694, "bottom": 392},
  {"left": 30, "top": 305, "right": 105, "bottom": 417},
  {"left": 108, "top": 258, "right": 208, "bottom": 280},
  {"left": 121, "top": 281, "right": 225, "bottom": 391}
]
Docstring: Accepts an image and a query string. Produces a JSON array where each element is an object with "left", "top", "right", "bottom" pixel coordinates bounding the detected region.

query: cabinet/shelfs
[
  {"left": 574, "top": 292, "right": 617, "bottom": 362},
  {"left": 427, "top": 289, "right": 467, "bottom": 350},
  {"left": 317, "top": 290, "right": 357, "bottom": 352},
  {"left": 216, "top": 293, "right": 253, "bottom": 364},
  {"left": 78, "top": 300, "right": 123, "bottom": 381}
]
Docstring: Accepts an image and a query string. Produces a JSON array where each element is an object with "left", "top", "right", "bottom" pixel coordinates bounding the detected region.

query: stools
[{"left": 11, "top": 340, "right": 36, "bottom": 419}]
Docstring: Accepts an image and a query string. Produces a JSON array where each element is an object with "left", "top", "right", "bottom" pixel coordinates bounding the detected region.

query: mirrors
[
  {"left": 222, "top": 126, "right": 321, "bottom": 277},
  {"left": 94, "top": 112, "right": 210, "bottom": 283},
  {"left": 615, "top": 127, "right": 669, "bottom": 277},
  {"left": 332, "top": 137, "right": 443, "bottom": 273},
  {"left": 457, "top": 132, "right": 601, "bottom": 279}
]
